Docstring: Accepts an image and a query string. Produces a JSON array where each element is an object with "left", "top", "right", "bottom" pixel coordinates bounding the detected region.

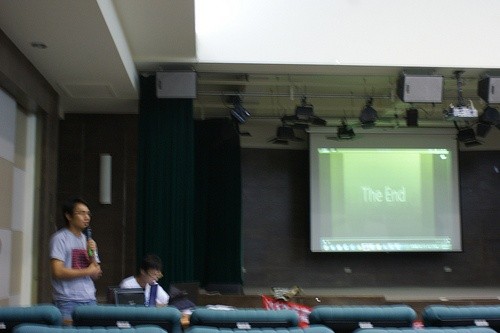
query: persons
[
  {"left": 49, "top": 200, "right": 102, "bottom": 325},
  {"left": 119, "top": 256, "right": 170, "bottom": 307}
]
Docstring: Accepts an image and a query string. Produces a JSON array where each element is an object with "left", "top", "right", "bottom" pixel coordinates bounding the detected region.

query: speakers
[
  {"left": 479, "top": 75, "right": 500, "bottom": 104},
  {"left": 154, "top": 69, "right": 198, "bottom": 100},
  {"left": 399, "top": 71, "right": 445, "bottom": 104}
]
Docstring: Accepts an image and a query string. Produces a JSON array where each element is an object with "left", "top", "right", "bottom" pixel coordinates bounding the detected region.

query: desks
[{"left": 197, "top": 294, "right": 386, "bottom": 308}]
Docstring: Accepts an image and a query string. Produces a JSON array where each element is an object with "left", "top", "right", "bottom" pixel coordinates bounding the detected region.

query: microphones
[{"left": 86, "top": 226, "right": 93, "bottom": 257}]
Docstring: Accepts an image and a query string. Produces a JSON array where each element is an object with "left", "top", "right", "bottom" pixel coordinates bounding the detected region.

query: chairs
[{"left": 0, "top": 297, "right": 500, "bottom": 332}]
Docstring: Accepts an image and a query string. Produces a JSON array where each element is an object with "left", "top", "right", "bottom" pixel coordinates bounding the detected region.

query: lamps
[{"left": 230, "top": 95, "right": 500, "bottom": 148}]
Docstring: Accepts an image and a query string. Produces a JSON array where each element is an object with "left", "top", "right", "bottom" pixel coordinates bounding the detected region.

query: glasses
[{"left": 70, "top": 211, "right": 91, "bottom": 216}]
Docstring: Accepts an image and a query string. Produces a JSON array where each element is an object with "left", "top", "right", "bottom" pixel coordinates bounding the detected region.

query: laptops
[
  {"left": 106, "top": 285, "right": 146, "bottom": 305},
  {"left": 168, "top": 282, "right": 199, "bottom": 304}
]
